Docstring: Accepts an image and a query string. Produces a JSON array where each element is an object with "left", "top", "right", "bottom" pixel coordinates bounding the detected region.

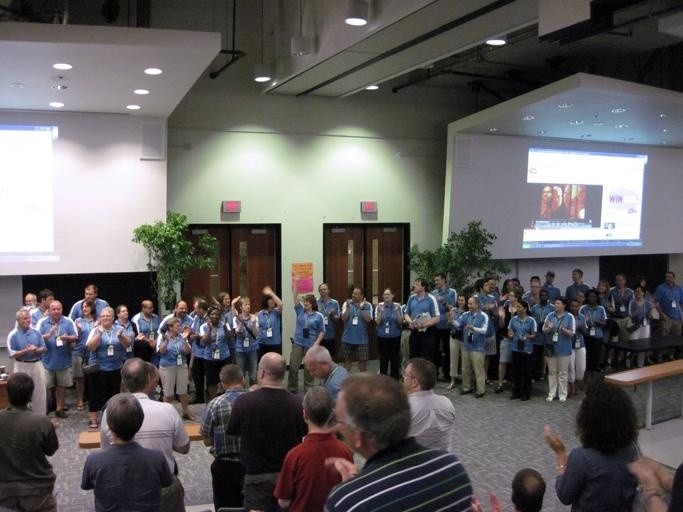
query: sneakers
[
  {"left": 88, "top": 417, "right": 98, "bottom": 429},
  {"left": 181, "top": 412, "right": 198, "bottom": 422},
  {"left": 54, "top": 409, "right": 68, "bottom": 419},
  {"left": 447, "top": 372, "right": 578, "bottom": 403}
]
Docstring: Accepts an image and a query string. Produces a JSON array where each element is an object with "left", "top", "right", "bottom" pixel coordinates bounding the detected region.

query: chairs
[{"left": 584, "top": 318, "right": 621, "bottom": 398}]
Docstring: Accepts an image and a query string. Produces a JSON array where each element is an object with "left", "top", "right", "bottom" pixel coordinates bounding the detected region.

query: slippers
[{"left": 76, "top": 397, "right": 85, "bottom": 412}]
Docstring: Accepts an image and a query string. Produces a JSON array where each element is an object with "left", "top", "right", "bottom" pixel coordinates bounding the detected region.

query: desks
[
  {"left": 609, "top": 334, "right": 682, "bottom": 393},
  {"left": 603, "top": 356, "right": 682, "bottom": 432},
  {"left": 77, "top": 424, "right": 209, "bottom": 450}
]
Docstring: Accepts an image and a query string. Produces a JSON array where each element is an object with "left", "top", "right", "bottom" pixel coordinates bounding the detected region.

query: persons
[
  {"left": 324, "top": 372, "right": 475, "bottom": 510},
  {"left": 375, "top": 287, "right": 404, "bottom": 380},
  {"left": 471, "top": 468, "right": 546, "bottom": 510},
  {"left": 400, "top": 358, "right": 456, "bottom": 452},
  {"left": 339, "top": 286, "right": 373, "bottom": 373},
  {"left": 288, "top": 271, "right": 325, "bottom": 394},
  {"left": 273, "top": 385, "right": 354, "bottom": 509},
  {"left": 542, "top": 185, "right": 585, "bottom": 217},
  {"left": 304, "top": 346, "right": 347, "bottom": 396},
  {"left": 402, "top": 269, "right": 681, "bottom": 401},
  {"left": 544, "top": 381, "right": 682, "bottom": 509},
  {"left": 1, "top": 372, "right": 60, "bottom": 509},
  {"left": 7, "top": 283, "right": 284, "bottom": 429},
  {"left": 226, "top": 352, "right": 308, "bottom": 509},
  {"left": 99, "top": 358, "right": 190, "bottom": 509},
  {"left": 80, "top": 392, "right": 173, "bottom": 508},
  {"left": 200, "top": 363, "right": 251, "bottom": 509},
  {"left": 316, "top": 283, "right": 341, "bottom": 362}
]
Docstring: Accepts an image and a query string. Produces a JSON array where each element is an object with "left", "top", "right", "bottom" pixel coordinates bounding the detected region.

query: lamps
[
  {"left": 343, "top": 1, "right": 370, "bottom": 27},
  {"left": 485, "top": 33, "right": 508, "bottom": 46},
  {"left": 290, "top": 1, "right": 312, "bottom": 58},
  {"left": 251, "top": 1, "right": 273, "bottom": 84}
]
